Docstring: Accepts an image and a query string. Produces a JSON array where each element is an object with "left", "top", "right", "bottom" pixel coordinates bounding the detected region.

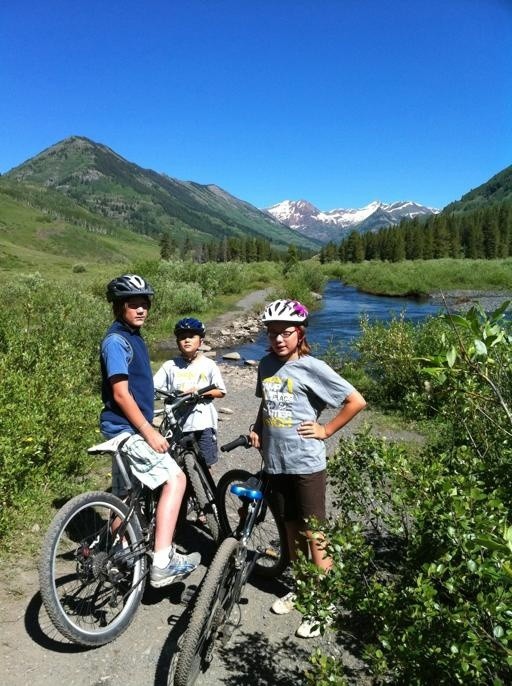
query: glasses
[{"left": 266, "top": 327, "right": 296, "bottom": 338}]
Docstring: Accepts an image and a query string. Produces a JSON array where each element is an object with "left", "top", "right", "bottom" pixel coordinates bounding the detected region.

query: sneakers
[
  {"left": 151, "top": 549, "right": 201, "bottom": 588},
  {"left": 272, "top": 584, "right": 312, "bottom": 616},
  {"left": 296, "top": 603, "right": 340, "bottom": 639}
]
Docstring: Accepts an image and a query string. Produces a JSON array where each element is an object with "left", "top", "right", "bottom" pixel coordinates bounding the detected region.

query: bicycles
[
  {"left": 39, "top": 386, "right": 217, "bottom": 648},
  {"left": 174, "top": 437, "right": 289, "bottom": 686}
]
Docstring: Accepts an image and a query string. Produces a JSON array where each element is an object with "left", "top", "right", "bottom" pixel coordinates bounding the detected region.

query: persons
[
  {"left": 152, "top": 318, "right": 226, "bottom": 526},
  {"left": 248, "top": 299, "right": 367, "bottom": 639},
  {"left": 99, "top": 274, "right": 202, "bottom": 589}
]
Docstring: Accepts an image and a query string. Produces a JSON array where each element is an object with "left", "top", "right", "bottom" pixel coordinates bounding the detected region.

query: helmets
[
  {"left": 106, "top": 273, "right": 154, "bottom": 302},
  {"left": 175, "top": 317, "right": 205, "bottom": 336},
  {"left": 261, "top": 297, "right": 308, "bottom": 324}
]
